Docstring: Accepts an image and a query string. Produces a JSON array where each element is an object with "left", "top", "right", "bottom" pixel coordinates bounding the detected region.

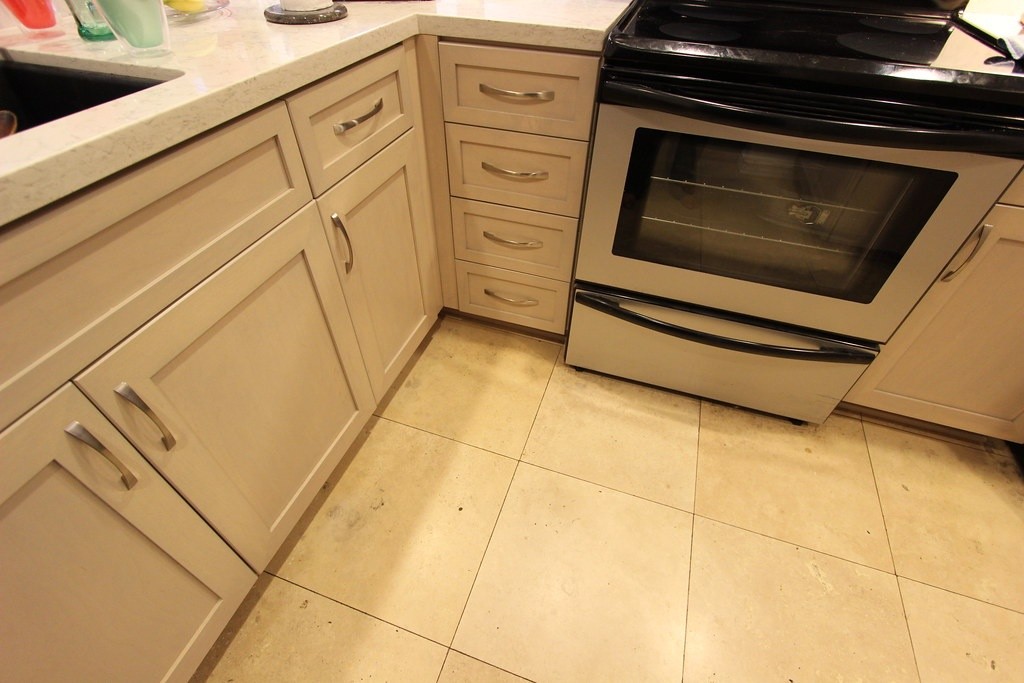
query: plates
[
  {"left": 0, "top": 110, "right": 17, "bottom": 138},
  {"left": 162, "top": 0, "right": 230, "bottom": 23}
]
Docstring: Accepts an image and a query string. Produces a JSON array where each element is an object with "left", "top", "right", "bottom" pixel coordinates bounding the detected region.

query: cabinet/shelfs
[
  {"left": 835, "top": 167, "right": 1024, "bottom": 444},
  {"left": 1, "top": 40, "right": 445, "bottom": 683},
  {"left": 416, "top": 34, "right": 601, "bottom": 339}
]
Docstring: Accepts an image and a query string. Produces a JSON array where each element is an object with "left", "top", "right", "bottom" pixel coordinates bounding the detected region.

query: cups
[
  {"left": 65, "top": 0, "right": 116, "bottom": 40},
  {"left": 93, "top": 0, "right": 171, "bottom": 57},
  {"left": 0, "top": 0, "right": 64, "bottom": 40}
]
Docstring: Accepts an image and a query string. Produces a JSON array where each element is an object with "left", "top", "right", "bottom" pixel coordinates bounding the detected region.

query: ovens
[{"left": 563, "top": 65, "right": 1024, "bottom": 423}]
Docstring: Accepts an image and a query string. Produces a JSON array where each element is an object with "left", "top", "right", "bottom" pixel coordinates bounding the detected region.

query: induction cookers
[{"left": 604, "top": 0, "right": 1024, "bottom": 120}]
